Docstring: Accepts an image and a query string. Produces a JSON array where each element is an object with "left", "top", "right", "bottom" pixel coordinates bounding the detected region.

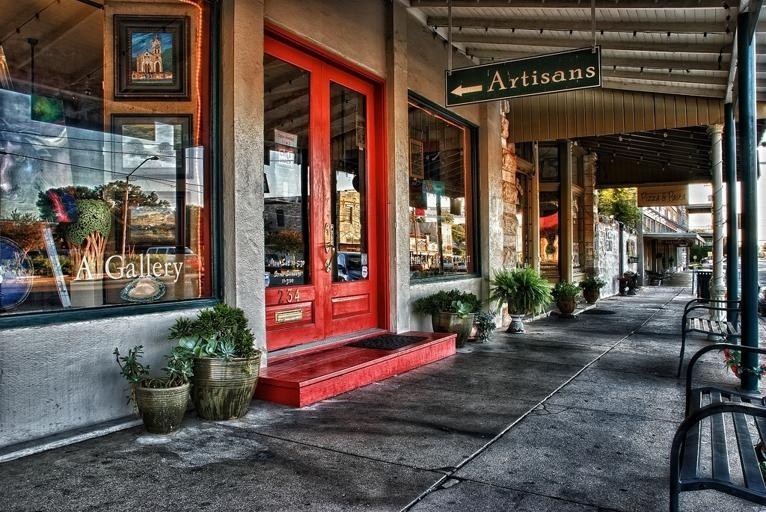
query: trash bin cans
[{"left": 695, "top": 270, "right": 713, "bottom": 299}]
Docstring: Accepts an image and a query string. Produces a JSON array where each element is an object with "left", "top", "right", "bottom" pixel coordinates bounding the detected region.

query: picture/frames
[
  {"left": 111, "top": 112, "right": 194, "bottom": 181},
  {"left": 110, "top": 12, "right": 191, "bottom": 101},
  {"left": 115, "top": 191, "right": 190, "bottom": 255}
]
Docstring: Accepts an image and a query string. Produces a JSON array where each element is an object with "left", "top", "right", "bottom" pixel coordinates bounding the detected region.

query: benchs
[
  {"left": 669, "top": 341, "right": 766, "bottom": 511},
  {"left": 674, "top": 286, "right": 759, "bottom": 376}
]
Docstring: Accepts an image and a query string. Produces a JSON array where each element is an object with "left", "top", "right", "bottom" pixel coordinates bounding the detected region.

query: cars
[{"left": 704, "top": 255, "right": 765, "bottom": 317}]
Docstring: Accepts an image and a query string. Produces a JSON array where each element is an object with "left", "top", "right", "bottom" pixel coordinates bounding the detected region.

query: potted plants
[
  {"left": 551, "top": 277, "right": 581, "bottom": 318},
  {"left": 481, "top": 262, "right": 556, "bottom": 335},
  {"left": 579, "top": 273, "right": 607, "bottom": 304},
  {"left": 113, "top": 343, "right": 196, "bottom": 431},
  {"left": 412, "top": 287, "right": 474, "bottom": 351},
  {"left": 167, "top": 301, "right": 262, "bottom": 420}
]
